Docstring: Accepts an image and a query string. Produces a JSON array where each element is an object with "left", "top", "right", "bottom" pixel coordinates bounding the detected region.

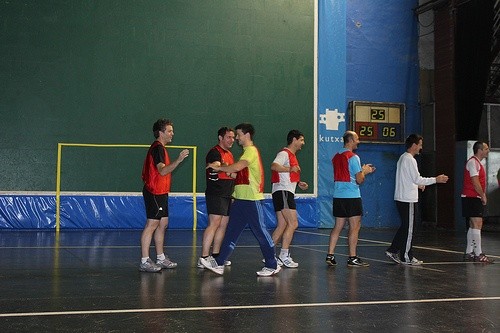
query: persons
[
  {"left": 199, "top": 123, "right": 281, "bottom": 277},
  {"left": 460, "top": 140, "right": 494, "bottom": 263},
  {"left": 262, "top": 129, "right": 308, "bottom": 268},
  {"left": 325, "top": 131, "right": 376, "bottom": 267},
  {"left": 197, "top": 126, "right": 237, "bottom": 269},
  {"left": 138, "top": 118, "right": 190, "bottom": 271},
  {"left": 384, "top": 133, "right": 449, "bottom": 266}
]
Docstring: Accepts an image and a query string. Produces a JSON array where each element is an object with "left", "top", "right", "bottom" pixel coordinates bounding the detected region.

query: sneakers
[
  {"left": 400, "top": 257, "right": 424, "bottom": 266},
  {"left": 326, "top": 254, "right": 337, "bottom": 266},
  {"left": 473, "top": 253, "right": 494, "bottom": 263},
  {"left": 261, "top": 253, "right": 283, "bottom": 266},
  {"left": 197, "top": 256, "right": 231, "bottom": 267},
  {"left": 257, "top": 265, "right": 281, "bottom": 276},
  {"left": 347, "top": 257, "right": 370, "bottom": 267},
  {"left": 384, "top": 250, "right": 401, "bottom": 264},
  {"left": 156, "top": 256, "right": 177, "bottom": 269},
  {"left": 464, "top": 252, "right": 474, "bottom": 260},
  {"left": 139, "top": 257, "right": 162, "bottom": 272},
  {"left": 201, "top": 257, "right": 224, "bottom": 275},
  {"left": 278, "top": 253, "right": 298, "bottom": 267}
]
